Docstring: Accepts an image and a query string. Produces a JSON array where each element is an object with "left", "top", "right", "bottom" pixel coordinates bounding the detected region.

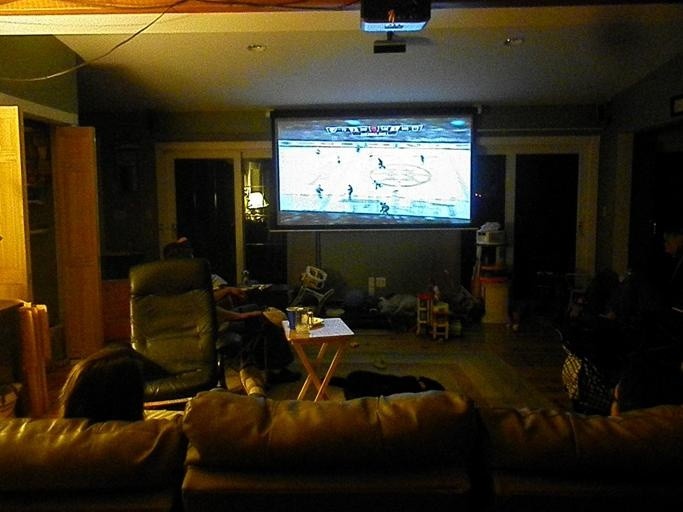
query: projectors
[{"left": 358, "top": 2, "right": 431, "bottom": 32}]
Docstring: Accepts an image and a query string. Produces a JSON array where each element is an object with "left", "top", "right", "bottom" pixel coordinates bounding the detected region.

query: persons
[
  {"left": 315, "top": 183, "right": 324, "bottom": 200},
  {"left": 419, "top": 154, "right": 425, "bottom": 164},
  {"left": 345, "top": 183, "right": 353, "bottom": 200},
  {"left": 378, "top": 200, "right": 389, "bottom": 216},
  {"left": 335, "top": 155, "right": 341, "bottom": 166},
  {"left": 370, "top": 178, "right": 383, "bottom": 191},
  {"left": 354, "top": 143, "right": 360, "bottom": 153},
  {"left": 160, "top": 237, "right": 303, "bottom": 387},
  {"left": 314, "top": 146, "right": 321, "bottom": 160},
  {"left": 55, "top": 341, "right": 267, "bottom": 425},
  {"left": 376, "top": 157, "right": 386, "bottom": 170}
]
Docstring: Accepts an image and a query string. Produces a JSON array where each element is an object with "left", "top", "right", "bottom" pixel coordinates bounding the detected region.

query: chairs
[
  {"left": 0, "top": 390, "right": 682, "bottom": 511},
  {"left": 127, "top": 258, "right": 226, "bottom": 410}
]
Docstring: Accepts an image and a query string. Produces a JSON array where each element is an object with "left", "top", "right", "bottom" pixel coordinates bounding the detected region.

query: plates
[{"left": 302, "top": 317, "right": 324, "bottom": 325}]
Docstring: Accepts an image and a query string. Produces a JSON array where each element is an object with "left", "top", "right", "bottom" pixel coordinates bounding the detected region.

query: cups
[
  {"left": 286, "top": 306, "right": 302, "bottom": 330},
  {"left": 295, "top": 307, "right": 314, "bottom": 334}
]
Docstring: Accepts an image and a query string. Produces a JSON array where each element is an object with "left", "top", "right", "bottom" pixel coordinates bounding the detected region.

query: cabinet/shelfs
[{"left": 0, "top": 92, "right": 104, "bottom": 374}]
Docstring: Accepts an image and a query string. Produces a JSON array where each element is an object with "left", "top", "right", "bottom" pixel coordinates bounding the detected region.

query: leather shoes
[{"left": 267, "top": 369, "right": 301, "bottom": 385}]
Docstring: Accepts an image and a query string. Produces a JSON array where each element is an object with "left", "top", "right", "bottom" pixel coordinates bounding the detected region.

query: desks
[{"left": 281, "top": 317, "right": 355, "bottom": 402}]
